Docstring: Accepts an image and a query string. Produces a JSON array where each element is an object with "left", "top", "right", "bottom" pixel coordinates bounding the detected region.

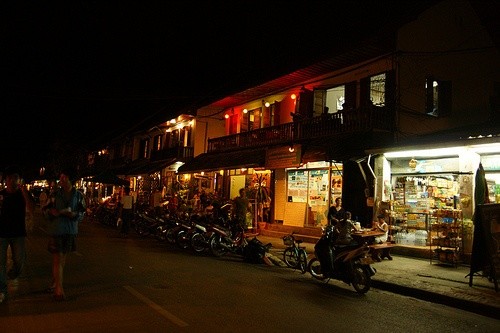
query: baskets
[{"left": 283, "top": 237, "right": 292, "bottom": 245}]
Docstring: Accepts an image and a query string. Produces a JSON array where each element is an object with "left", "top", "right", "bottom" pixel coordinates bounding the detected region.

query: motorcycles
[
  {"left": 136, "top": 206, "right": 191, "bottom": 247},
  {"left": 191, "top": 218, "right": 224, "bottom": 252},
  {"left": 208, "top": 218, "right": 249, "bottom": 257},
  {"left": 308, "top": 219, "right": 376, "bottom": 294}
]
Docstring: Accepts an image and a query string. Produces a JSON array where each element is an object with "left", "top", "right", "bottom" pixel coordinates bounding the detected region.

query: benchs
[{"left": 369, "top": 242, "right": 403, "bottom": 261}]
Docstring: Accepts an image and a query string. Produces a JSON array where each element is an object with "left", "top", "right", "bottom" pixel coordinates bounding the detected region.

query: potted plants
[{"left": 245, "top": 186, "right": 268, "bottom": 230}]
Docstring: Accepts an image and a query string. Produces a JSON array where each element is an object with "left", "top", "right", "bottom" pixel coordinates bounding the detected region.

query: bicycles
[{"left": 280, "top": 231, "right": 307, "bottom": 274}]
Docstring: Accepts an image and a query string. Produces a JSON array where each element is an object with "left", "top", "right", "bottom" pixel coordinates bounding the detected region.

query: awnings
[
  {"left": 34, "top": 157, "right": 181, "bottom": 185},
  {"left": 178, "top": 151, "right": 265, "bottom": 174}
]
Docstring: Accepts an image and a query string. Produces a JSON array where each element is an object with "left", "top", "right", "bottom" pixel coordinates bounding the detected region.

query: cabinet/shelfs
[{"left": 395, "top": 176, "right": 495, "bottom": 270}]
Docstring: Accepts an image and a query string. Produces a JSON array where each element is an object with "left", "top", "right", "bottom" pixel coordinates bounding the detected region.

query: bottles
[{"left": 389, "top": 201, "right": 428, "bottom": 246}]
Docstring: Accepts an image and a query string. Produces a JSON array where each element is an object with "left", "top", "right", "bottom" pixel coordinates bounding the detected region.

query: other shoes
[
  {"left": 49, "top": 280, "right": 65, "bottom": 301},
  {"left": 0, "top": 290, "right": 10, "bottom": 305},
  {"left": 11, "top": 276, "right": 22, "bottom": 288}
]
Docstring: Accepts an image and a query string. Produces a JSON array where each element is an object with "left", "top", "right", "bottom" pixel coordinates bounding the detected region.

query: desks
[{"left": 351, "top": 231, "right": 386, "bottom": 245}]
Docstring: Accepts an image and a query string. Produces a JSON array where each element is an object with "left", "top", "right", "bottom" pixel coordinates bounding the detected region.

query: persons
[
  {"left": 0, "top": 170, "right": 432, "bottom": 302},
  {"left": 289, "top": 103, "right": 348, "bottom": 126}
]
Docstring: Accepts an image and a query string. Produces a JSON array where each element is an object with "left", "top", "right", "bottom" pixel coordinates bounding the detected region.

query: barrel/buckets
[{"left": 258, "top": 222, "right": 266, "bottom": 229}]
